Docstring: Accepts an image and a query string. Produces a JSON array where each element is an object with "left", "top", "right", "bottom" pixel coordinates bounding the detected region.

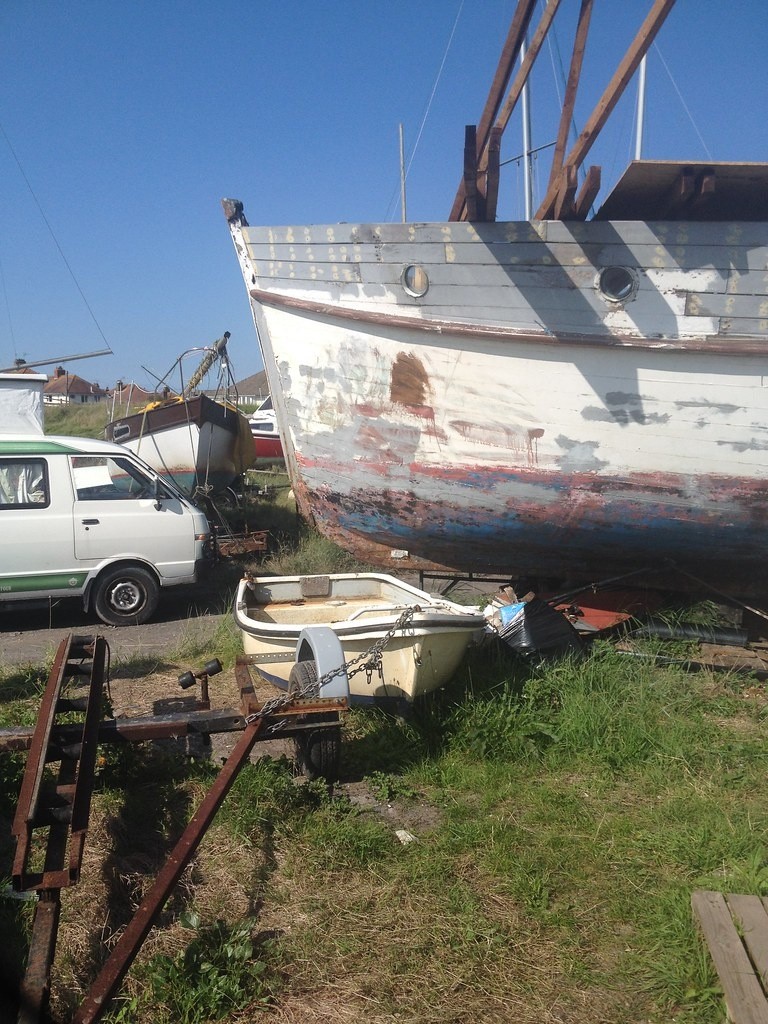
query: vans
[{"left": 0, "top": 436, "right": 213, "bottom": 629}]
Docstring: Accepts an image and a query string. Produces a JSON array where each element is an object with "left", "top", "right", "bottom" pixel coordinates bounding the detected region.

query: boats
[
  {"left": 234, "top": 569, "right": 486, "bottom": 713},
  {"left": 221, "top": 195, "right": 767, "bottom": 590},
  {"left": 101, "top": 394, "right": 254, "bottom": 507},
  {"left": 247, "top": 394, "right": 288, "bottom": 458}
]
